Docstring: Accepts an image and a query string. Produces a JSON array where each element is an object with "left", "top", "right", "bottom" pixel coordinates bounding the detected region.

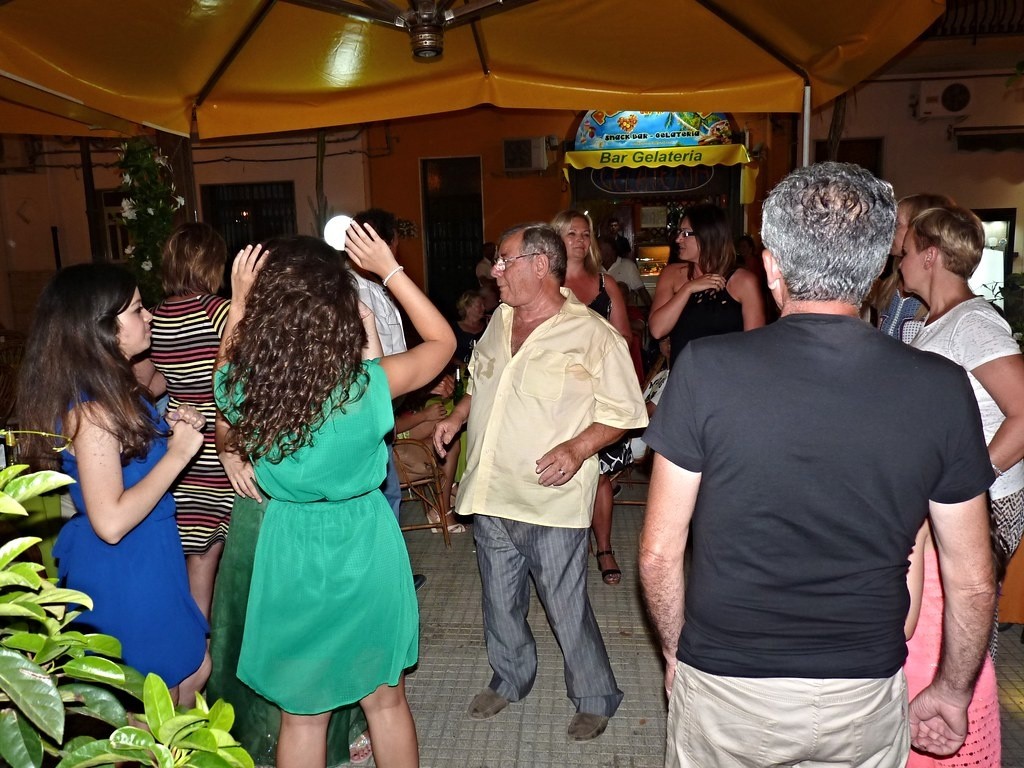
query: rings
[
  {"left": 559, "top": 469, "right": 566, "bottom": 476},
  {"left": 714, "top": 280, "right": 717, "bottom": 284}
]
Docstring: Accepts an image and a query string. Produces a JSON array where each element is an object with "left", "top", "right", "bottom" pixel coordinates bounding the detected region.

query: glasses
[
  {"left": 677, "top": 228, "right": 695, "bottom": 237},
  {"left": 494, "top": 253, "right": 550, "bottom": 272}
]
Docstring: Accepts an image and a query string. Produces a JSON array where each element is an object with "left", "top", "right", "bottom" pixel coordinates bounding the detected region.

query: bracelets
[
  {"left": 383, "top": 266, "right": 404, "bottom": 286},
  {"left": 991, "top": 463, "right": 1003, "bottom": 476}
]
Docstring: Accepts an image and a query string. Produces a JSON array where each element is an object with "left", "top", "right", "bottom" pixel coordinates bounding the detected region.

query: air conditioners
[{"left": 920, "top": 80, "right": 971, "bottom": 117}]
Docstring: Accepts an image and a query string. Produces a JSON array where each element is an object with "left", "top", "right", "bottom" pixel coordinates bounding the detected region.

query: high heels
[
  {"left": 450, "top": 482, "right": 457, "bottom": 507},
  {"left": 596, "top": 550, "right": 621, "bottom": 585},
  {"left": 427, "top": 507, "right": 466, "bottom": 533}
]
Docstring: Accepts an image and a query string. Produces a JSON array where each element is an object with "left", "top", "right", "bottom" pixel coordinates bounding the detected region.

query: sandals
[{"left": 349, "top": 734, "right": 372, "bottom": 764}]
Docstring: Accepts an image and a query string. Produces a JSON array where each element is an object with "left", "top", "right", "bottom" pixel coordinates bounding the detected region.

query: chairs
[{"left": 392, "top": 439, "right": 452, "bottom": 551}]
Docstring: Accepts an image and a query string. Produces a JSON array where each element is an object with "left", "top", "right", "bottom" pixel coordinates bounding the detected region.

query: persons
[
  {"left": 148, "top": 203, "right": 772, "bottom": 767},
  {"left": 10, "top": 260, "right": 213, "bottom": 768},
  {"left": 639, "top": 160, "right": 997, "bottom": 768},
  {"left": 880, "top": 191, "right": 1024, "bottom": 768}
]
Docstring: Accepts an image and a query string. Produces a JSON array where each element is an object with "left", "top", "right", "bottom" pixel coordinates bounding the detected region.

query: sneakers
[
  {"left": 568, "top": 713, "right": 609, "bottom": 745},
  {"left": 467, "top": 687, "right": 509, "bottom": 722}
]
